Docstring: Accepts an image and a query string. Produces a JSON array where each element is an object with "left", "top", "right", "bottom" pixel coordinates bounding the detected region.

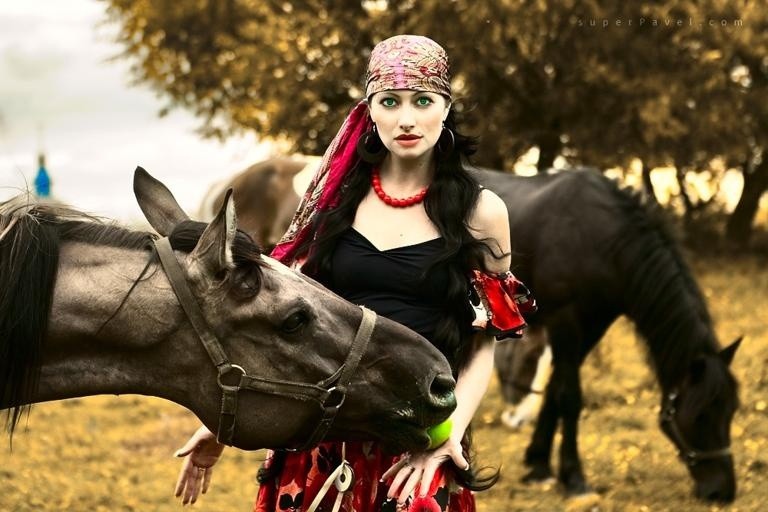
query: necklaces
[{"left": 371, "top": 164, "right": 428, "bottom": 208}]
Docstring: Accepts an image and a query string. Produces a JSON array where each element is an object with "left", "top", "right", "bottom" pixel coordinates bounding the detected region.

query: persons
[{"left": 173, "top": 34, "right": 512, "bottom": 512}]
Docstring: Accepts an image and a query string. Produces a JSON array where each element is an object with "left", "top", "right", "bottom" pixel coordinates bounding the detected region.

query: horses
[
  {"left": 211, "top": 154, "right": 553, "bottom": 432},
  {"left": 0, "top": 167, "right": 458, "bottom": 452},
  {"left": 461, "top": 163, "right": 743, "bottom": 512}
]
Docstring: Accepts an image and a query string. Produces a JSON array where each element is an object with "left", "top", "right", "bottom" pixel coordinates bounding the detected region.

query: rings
[
  {"left": 198, "top": 467, "right": 207, "bottom": 471},
  {"left": 406, "top": 464, "right": 413, "bottom": 470}
]
